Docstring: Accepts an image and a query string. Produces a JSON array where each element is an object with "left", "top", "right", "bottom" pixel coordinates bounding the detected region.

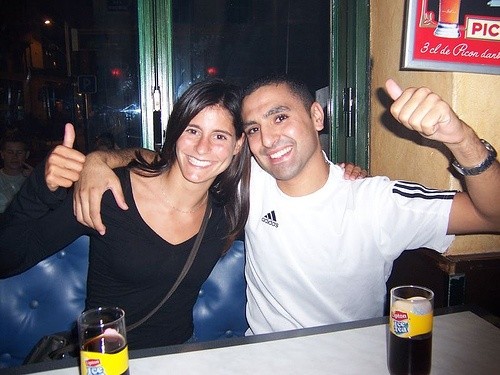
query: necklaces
[{"left": 159, "top": 174, "right": 209, "bottom": 214}]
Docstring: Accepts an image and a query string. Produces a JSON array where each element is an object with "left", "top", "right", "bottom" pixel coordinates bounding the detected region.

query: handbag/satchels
[{"left": 21, "top": 329, "right": 80, "bottom": 366}]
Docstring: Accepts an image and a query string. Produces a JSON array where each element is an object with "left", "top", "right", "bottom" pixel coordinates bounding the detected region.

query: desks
[{"left": 0, "top": 303, "right": 500, "bottom": 375}]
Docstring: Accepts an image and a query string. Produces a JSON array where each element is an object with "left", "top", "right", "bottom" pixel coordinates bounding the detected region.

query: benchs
[{"left": 0, "top": 235, "right": 249, "bottom": 370}]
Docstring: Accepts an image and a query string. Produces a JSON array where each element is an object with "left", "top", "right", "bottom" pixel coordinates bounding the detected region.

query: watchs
[{"left": 451, "top": 139, "right": 498, "bottom": 176}]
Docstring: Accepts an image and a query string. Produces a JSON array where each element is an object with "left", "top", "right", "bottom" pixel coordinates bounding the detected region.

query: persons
[
  {"left": 0, "top": 131, "right": 37, "bottom": 215},
  {"left": 95, "top": 131, "right": 115, "bottom": 152},
  {"left": 72, "top": 73, "right": 500, "bottom": 337},
  {"left": 0, "top": 78, "right": 369, "bottom": 358}
]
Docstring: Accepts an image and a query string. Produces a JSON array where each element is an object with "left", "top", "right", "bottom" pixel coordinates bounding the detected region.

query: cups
[
  {"left": 389, "top": 286, "right": 434, "bottom": 375},
  {"left": 433, "top": 0, "right": 460, "bottom": 38},
  {"left": 78, "top": 307, "right": 129, "bottom": 375}
]
